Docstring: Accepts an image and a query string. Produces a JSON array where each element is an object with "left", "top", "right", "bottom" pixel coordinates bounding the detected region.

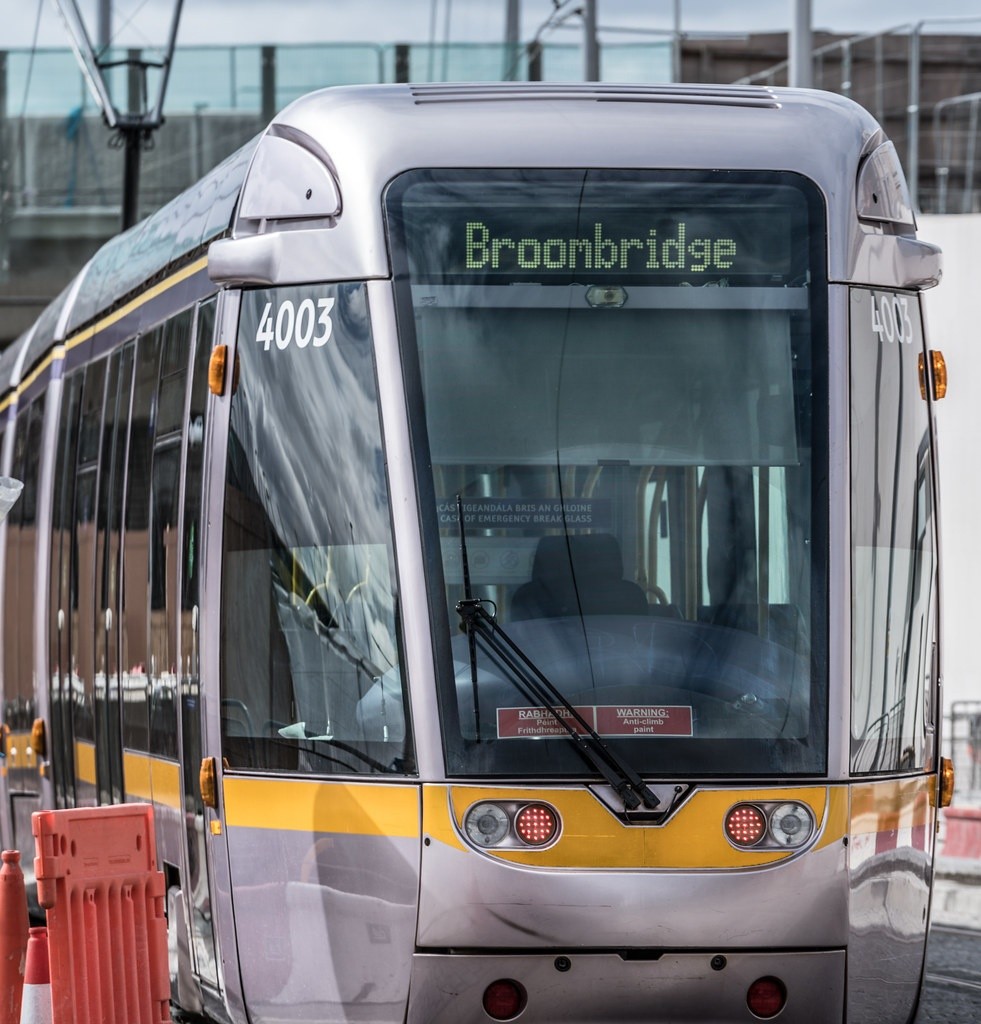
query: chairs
[{"left": 513, "top": 533, "right": 649, "bottom": 624}]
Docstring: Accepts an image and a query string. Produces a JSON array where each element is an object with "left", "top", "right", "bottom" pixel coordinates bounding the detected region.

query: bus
[{"left": 0, "top": 82, "right": 960, "bottom": 1024}]
